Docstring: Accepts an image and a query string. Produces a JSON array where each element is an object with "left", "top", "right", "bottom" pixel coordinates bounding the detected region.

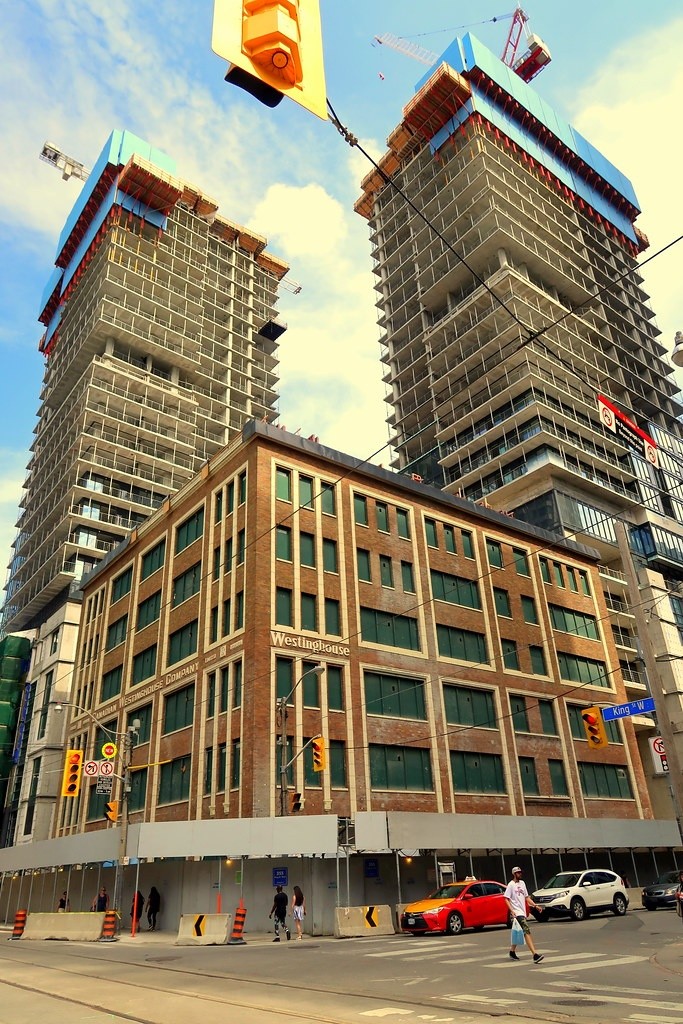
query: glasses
[{"left": 102, "top": 889, "right": 106, "bottom": 891}]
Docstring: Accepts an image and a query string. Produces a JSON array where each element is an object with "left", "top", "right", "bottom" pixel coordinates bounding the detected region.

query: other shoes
[
  {"left": 137, "top": 927, "right": 140, "bottom": 932},
  {"left": 509, "top": 951, "right": 519, "bottom": 960},
  {"left": 147, "top": 925, "right": 153, "bottom": 931},
  {"left": 533, "top": 954, "right": 544, "bottom": 963},
  {"left": 150, "top": 928, "right": 155, "bottom": 932},
  {"left": 297, "top": 934, "right": 302, "bottom": 939},
  {"left": 273, "top": 938, "right": 280, "bottom": 942},
  {"left": 129, "top": 929, "right": 132, "bottom": 933},
  {"left": 287, "top": 932, "right": 291, "bottom": 940}
]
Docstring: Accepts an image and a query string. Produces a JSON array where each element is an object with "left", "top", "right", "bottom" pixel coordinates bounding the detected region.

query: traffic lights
[
  {"left": 104, "top": 800, "right": 119, "bottom": 822},
  {"left": 286, "top": 790, "right": 302, "bottom": 814},
  {"left": 60, "top": 749, "right": 83, "bottom": 798},
  {"left": 581, "top": 706, "right": 610, "bottom": 750},
  {"left": 310, "top": 737, "right": 325, "bottom": 773}
]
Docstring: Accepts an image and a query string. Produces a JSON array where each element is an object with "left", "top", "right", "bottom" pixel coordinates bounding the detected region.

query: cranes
[{"left": 371, "top": 1, "right": 551, "bottom": 85}]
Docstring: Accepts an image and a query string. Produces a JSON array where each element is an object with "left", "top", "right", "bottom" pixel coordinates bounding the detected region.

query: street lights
[
  {"left": 281, "top": 665, "right": 325, "bottom": 819},
  {"left": 54, "top": 703, "right": 131, "bottom": 936}
]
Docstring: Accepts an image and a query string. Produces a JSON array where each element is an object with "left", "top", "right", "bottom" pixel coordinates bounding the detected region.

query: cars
[
  {"left": 400, "top": 876, "right": 530, "bottom": 937},
  {"left": 641, "top": 870, "right": 683, "bottom": 911}
]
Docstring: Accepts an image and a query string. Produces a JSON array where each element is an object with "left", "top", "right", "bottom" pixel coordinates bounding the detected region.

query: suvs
[{"left": 529, "top": 868, "right": 630, "bottom": 922}]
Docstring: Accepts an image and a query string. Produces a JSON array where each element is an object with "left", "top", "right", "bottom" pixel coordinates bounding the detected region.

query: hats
[{"left": 511, "top": 867, "right": 521, "bottom": 875}]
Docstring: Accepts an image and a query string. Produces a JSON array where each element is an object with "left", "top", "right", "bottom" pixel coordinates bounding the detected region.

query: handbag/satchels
[{"left": 511, "top": 918, "right": 527, "bottom": 945}]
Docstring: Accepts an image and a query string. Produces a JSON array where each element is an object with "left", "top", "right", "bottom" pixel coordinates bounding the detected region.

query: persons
[
  {"left": 56, "top": 891, "right": 69, "bottom": 912},
  {"left": 93, "top": 886, "right": 109, "bottom": 912},
  {"left": 130, "top": 890, "right": 145, "bottom": 932},
  {"left": 145, "top": 887, "right": 160, "bottom": 932},
  {"left": 269, "top": 885, "right": 291, "bottom": 942},
  {"left": 289, "top": 886, "right": 306, "bottom": 940},
  {"left": 504, "top": 867, "right": 544, "bottom": 963}
]
